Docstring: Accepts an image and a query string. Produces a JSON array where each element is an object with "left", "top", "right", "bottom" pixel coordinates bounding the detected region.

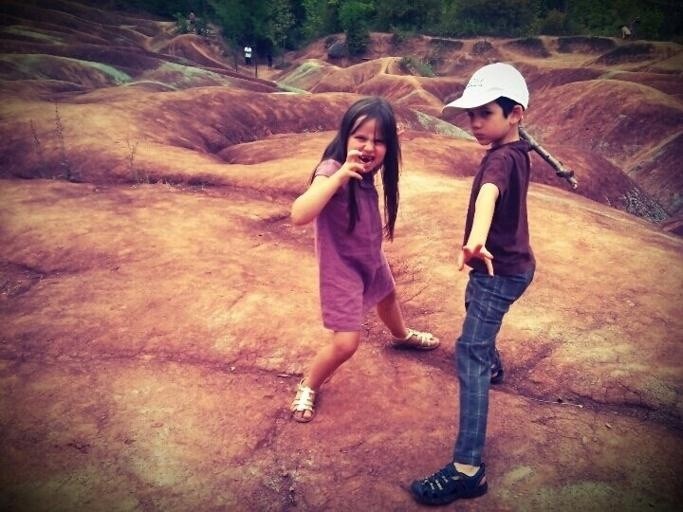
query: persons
[
  {"left": 244, "top": 43, "right": 252, "bottom": 65},
  {"left": 411, "top": 61, "right": 536, "bottom": 505},
  {"left": 618, "top": 24, "right": 632, "bottom": 39},
  {"left": 189, "top": 10, "right": 196, "bottom": 24},
  {"left": 287, "top": 96, "right": 440, "bottom": 424},
  {"left": 267, "top": 50, "right": 272, "bottom": 68}
]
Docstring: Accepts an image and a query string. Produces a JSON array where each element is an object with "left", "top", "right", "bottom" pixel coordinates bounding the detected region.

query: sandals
[
  {"left": 290, "top": 381, "right": 315, "bottom": 423},
  {"left": 410, "top": 462, "right": 489, "bottom": 506},
  {"left": 390, "top": 327, "right": 440, "bottom": 350},
  {"left": 490, "top": 348, "right": 504, "bottom": 385}
]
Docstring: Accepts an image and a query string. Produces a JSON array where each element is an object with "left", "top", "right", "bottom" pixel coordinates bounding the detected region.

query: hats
[{"left": 442, "top": 63, "right": 528, "bottom": 117}]
